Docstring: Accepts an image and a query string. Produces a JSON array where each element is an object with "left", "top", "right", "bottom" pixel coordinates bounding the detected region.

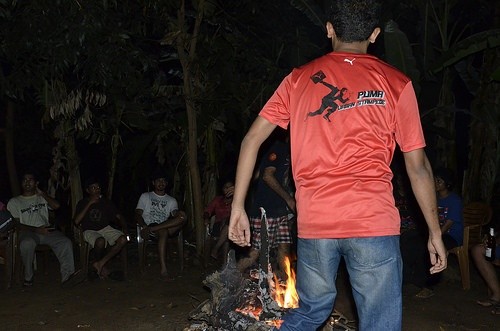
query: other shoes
[
  {"left": 416, "top": 290, "right": 435, "bottom": 298},
  {"left": 62, "top": 269, "right": 86, "bottom": 289},
  {"left": 158, "top": 271, "right": 175, "bottom": 283},
  {"left": 24, "top": 277, "right": 33, "bottom": 289},
  {"left": 110, "top": 270, "right": 123, "bottom": 281},
  {"left": 208, "top": 254, "right": 217, "bottom": 266},
  {"left": 88, "top": 270, "right": 97, "bottom": 281}
]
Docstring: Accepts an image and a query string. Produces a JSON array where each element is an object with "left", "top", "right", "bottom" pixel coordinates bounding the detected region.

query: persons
[
  {"left": 203, "top": 182, "right": 235, "bottom": 269},
  {"left": 236, "top": 124, "right": 295, "bottom": 282},
  {"left": 228, "top": 0, "right": 448, "bottom": 331},
  {"left": 72, "top": 180, "right": 129, "bottom": 280},
  {"left": 136, "top": 173, "right": 187, "bottom": 281},
  {"left": 5, "top": 173, "right": 75, "bottom": 289},
  {"left": 393, "top": 115, "right": 500, "bottom": 316}
]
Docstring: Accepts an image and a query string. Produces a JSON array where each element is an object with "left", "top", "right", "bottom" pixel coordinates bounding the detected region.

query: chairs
[
  {"left": 442, "top": 201, "right": 492, "bottom": 291},
  {"left": 5, "top": 211, "right": 207, "bottom": 289}
]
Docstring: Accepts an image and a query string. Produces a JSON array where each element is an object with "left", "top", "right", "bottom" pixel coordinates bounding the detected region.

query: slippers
[{"left": 475, "top": 298, "right": 500, "bottom": 316}]
